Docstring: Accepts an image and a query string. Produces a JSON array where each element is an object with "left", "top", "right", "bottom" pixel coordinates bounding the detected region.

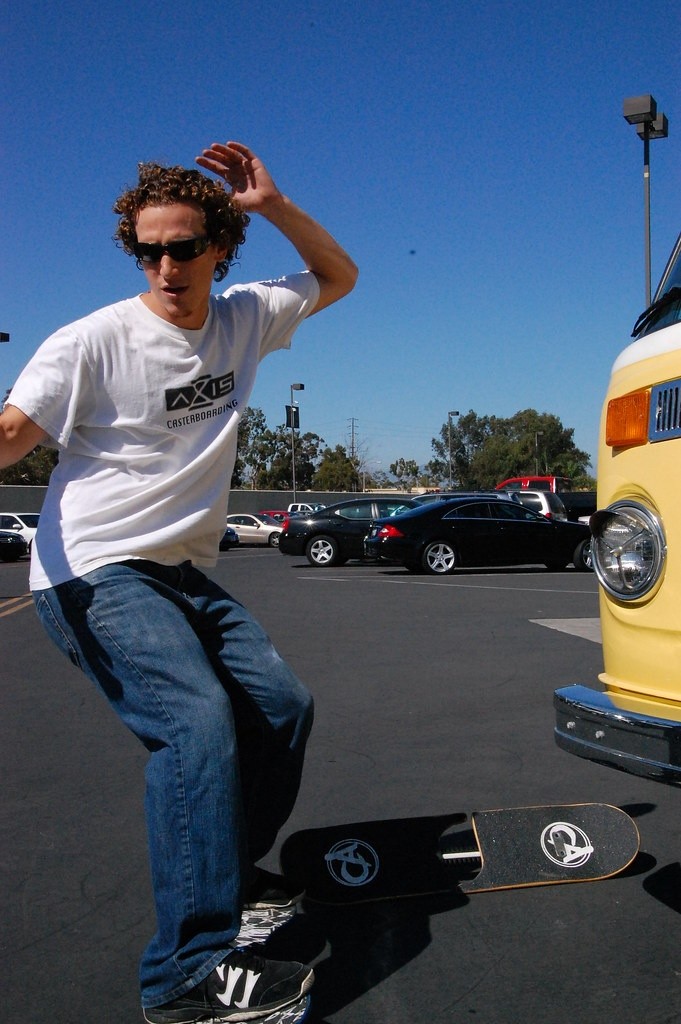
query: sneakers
[
  {"left": 143, "top": 948, "right": 314, "bottom": 1024},
  {"left": 234, "top": 864, "right": 306, "bottom": 908}
]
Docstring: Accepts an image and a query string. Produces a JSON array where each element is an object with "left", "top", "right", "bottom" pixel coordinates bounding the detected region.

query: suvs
[
  {"left": 505, "top": 490, "right": 567, "bottom": 521},
  {"left": 389, "top": 491, "right": 525, "bottom": 518}
]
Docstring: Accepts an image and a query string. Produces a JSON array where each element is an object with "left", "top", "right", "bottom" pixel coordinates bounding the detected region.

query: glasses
[{"left": 128, "top": 230, "right": 211, "bottom": 262}]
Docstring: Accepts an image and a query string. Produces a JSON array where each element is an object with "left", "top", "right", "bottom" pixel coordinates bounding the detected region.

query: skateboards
[
  {"left": 280, "top": 799, "right": 644, "bottom": 908},
  {"left": 199, "top": 913, "right": 315, "bottom": 1024}
]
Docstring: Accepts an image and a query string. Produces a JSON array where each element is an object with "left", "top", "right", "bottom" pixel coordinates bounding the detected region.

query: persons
[{"left": 0, "top": 141, "right": 360, "bottom": 1024}]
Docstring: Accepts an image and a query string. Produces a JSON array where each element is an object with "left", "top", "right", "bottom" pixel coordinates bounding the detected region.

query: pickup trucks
[
  {"left": 495, "top": 476, "right": 597, "bottom": 515},
  {"left": 275, "top": 503, "right": 325, "bottom": 515}
]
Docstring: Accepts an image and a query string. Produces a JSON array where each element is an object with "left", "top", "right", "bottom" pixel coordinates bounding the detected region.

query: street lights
[
  {"left": 448, "top": 411, "right": 459, "bottom": 489},
  {"left": 535, "top": 431, "right": 544, "bottom": 476},
  {"left": 622, "top": 93, "right": 668, "bottom": 307},
  {"left": 290, "top": 383, "right": 305, "bottom": 503},
  {"left": 363, "top": 461, "right": 382, "bottom": 492}
]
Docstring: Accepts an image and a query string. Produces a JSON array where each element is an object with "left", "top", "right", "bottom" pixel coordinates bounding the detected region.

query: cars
[
  {"left": 255, "top": 511, "right": 304, "bottom": 526},
  {"left": 227, "top": 513, "right": 283, "bottom": 548},
  {"left": 0, "top": 513, "right": 40, "bottom": 553},
  {"left": 277, "top": 497, "right": 425, "bottom": 566},
  {"left": 0, "top": 531, "right": 28, "bottom": 562},
  {"left": 364, "top": 497, "right": 596, "bottom": 574},
  {"left": 219, "top": 527, "right": 240, "bottom": 551}
]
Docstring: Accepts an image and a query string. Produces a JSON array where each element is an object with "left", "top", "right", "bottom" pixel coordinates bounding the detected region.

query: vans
[{"left": 551, "top": 229, "right": 681, "bottom": 789}]
